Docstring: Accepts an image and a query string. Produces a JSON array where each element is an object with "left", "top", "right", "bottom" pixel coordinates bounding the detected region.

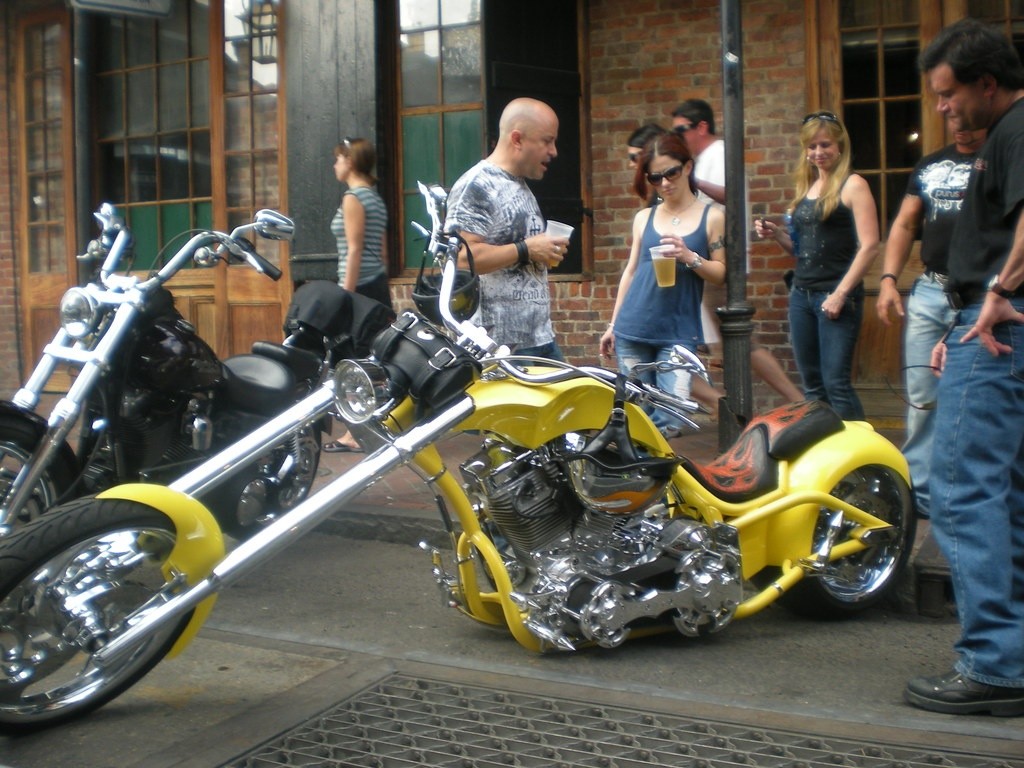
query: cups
[
  {"left": 648, "top": 244, "right": 676, "bottom": 288},
  {"left": 544, "top": 219, "right": 574, "bottom": 267}
]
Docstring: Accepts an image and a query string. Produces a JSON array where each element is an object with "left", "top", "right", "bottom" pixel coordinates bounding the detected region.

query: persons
[
  {"left": 318, "top": 136, "right": 393, "bottom": 454},
  {"left": 902, "top": 20, "right": 1024, "bottom": 719},
  {"left": 626, "top": 98, "right": 806, "bottom": 423},
  {"left": 442, "top": 98, "right": 569, "bottom": 367},
  {"left": 754, "top": 109, "right": 880, "bottom": 421},
  {"left": 599, "top": 132, "right": 726, "bottom": 440},
  {"left": 876, "top": 127, "right": 989, "bottom": 517}
]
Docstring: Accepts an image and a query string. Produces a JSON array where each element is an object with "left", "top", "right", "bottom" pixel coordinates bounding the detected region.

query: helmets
[
  {"left": 412, "top": 270, "right": 480, "bottom": 326},
  {"left": 551, "top": 437, "right": 685, "bottom": 515}
]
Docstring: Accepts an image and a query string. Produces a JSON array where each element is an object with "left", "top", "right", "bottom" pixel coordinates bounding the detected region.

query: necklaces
[{"left": 662, "top": 196, "right": 696, "bottom": 226}]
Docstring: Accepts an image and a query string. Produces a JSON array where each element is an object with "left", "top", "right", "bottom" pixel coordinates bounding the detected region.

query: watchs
[{"left": 988, "top": 273, "right": 1015, "bottom": 299}]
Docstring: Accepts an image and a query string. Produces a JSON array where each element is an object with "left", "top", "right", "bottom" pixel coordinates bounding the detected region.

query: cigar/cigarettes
[{"left": 600, "top": 353, "right": 611, "bottom": 357}]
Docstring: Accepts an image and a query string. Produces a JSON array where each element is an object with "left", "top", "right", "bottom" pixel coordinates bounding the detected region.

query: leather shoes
[{"left": 904, "top": 670, "right": 1024, "bottom": 717}]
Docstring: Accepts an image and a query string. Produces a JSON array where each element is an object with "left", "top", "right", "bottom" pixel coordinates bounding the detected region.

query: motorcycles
[
  {"left": 0, "top": 202, "right": 397, "bottom": 541},
  {"left": 0, "top": 182, "right": 914, "bottom": 736}
]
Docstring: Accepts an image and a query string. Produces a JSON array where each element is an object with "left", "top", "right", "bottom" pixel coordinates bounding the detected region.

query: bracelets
[
  {"left": 685, "top": 252, "right": 702, "bottom": 270},
  {"left": 880, "top": 273, "right": 897, "bottom": 284},
  {"left": 514, "top": 240, "right": 529, "bottom": 263},
  {"left": 608, "top": 323, "right": 615, "bottom": 326}
]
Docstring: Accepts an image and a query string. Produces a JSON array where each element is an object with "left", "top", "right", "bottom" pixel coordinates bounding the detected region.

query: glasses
[
  {"left": 645, "top": 162, "right": 685, "bottom": 186},
  {"left": 341, "top": 136, "right": 351, "bottom": 149},
  {"left": 673, "top": 123, "right": 696, "bottom": 133},
  {"left": 628, "top": 151, "right": 646, "bottom": 162},
  {"left": 802, "top": 112, "right": 842, "bottom": 130}
]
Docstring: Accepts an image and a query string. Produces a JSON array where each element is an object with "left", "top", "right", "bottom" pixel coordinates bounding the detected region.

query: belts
[
  {"left": 923, "top": 268, "right": 948, "bottom": 285},
  {"left": 946, "top": 290, "right": 989, "bottom": 310}
]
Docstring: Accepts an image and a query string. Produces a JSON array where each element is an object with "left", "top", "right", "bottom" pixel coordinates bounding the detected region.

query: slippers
[{"left": 322, "top": 440, "right": 363, "bottom": 453}]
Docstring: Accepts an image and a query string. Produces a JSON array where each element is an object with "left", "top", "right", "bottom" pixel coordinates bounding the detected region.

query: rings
[
  {"left": 821, "top": 308, "right": 825, "bottom": 312},
  {"left": 556, "top": 246, "right": 561, "bottom": 253}
]
